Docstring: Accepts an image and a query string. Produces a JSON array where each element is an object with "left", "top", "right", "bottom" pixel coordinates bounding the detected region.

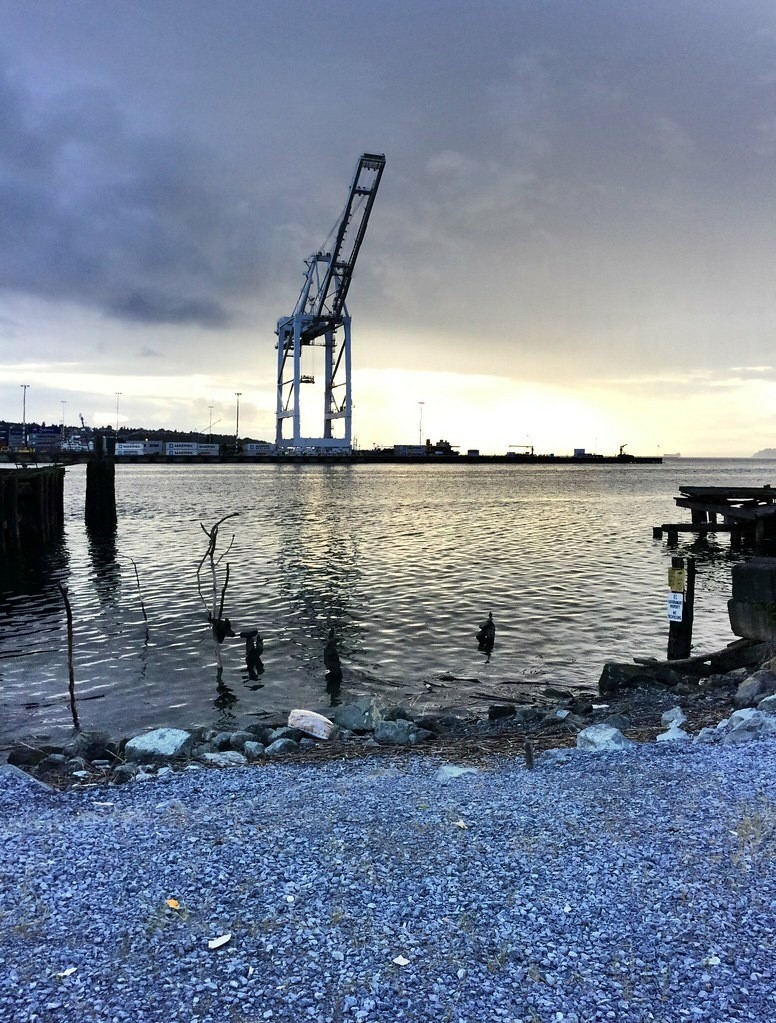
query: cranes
[{"left": 274, "top": 153, "right": 388, "bottom": 456}]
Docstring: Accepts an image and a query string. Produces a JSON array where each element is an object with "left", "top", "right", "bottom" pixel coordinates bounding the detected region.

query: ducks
[
  {"left": 324, "top": 627, "right": 339, "bottom": 645},
  {"left": 207, "top": 618, "right": 231, "bottom": 629},
  {"left": 479, "top": 611, "right": 495, "bottom": 631}
]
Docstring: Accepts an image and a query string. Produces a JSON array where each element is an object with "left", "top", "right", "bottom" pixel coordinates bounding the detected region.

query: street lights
[
  {"left": 209, "top": 406, "right": 213, "bottom": 444},
  {"left": 20, "top": 384, "right": 30, "bottom": 443},
  {"left": 418, "top": 402, "right": 425, "bottom": 446},
  {"left": 115, "top": 392, "right": 122, "bottom": 439},
  {"left": 61, "top": 400, "right": 67, "bottom": 440},
  {"left": 235, "top": 392, "right": 242, "bottom": 453}
]
